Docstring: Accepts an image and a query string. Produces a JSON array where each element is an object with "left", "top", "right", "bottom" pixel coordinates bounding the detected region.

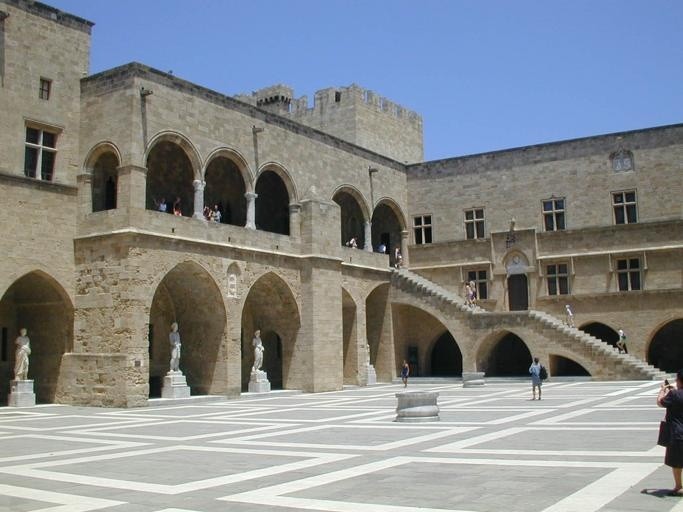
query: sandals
[{"left": 671, "top": 487, "right": 683, "bottom": 496}]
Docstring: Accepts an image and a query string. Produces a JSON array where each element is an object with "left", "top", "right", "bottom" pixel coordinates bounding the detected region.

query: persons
[
  {"left": 400, "top": 359, "right": 410, "bottom": 388},
  {"left": 467, "top": 275, "right": 477, "bottom": 307},
  {"left": 11, "top": 327, "right": 32, "bottom": 380},
  {"left": 213, "top": 204, "right": 222, "bottom": 223},
  {"left": 250, "top": 329, "right": 265, "bottom": 374},
  {"left": 169, "top": 321, "right": 183, "bottom": 373},
  {"left": 528, "top": 356, "right": 543, "bottom": 401},
  {"left": 345, "top": 235, "right": 353, "bottom": 248},
  {"left": 377, "top": 240, "right": 386, "bottom": 254},
  {"left": 394, "top": 243, "right": 403, "bottom": 270},
  {"left": 565, "top": 304, "right": 575, "bottom": 328},
  {"left": 349, "top": 233, "right": 358, "bottom": 249},
  {"left": 171, "top": 195, "right": 183, "bottom": 216},
  {"left": 615, "top": 329, "right": 626, "bottom": 355},
  {"left": 463, "top": 281, "right": 472, "bottom": 308},
  {"left": 656, "top": 368, "right": 682, "bottom": 497},
  {"left": 202, "top": 204, "right": 212, "bottom": 221},
  {"left": 149, "top": 193, "right": 168, "bottom": 212},
  {"left": 208, "top": 207, "right": 217, "bottom": 222}
]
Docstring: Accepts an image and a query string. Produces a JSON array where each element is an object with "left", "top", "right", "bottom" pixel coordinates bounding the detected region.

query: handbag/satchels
[
  {"left": 658, "top": 421, "right": 673, "bottom": 447},
  {"left": 540, "top": 364, "right": 547, "bottom": 379}
]
